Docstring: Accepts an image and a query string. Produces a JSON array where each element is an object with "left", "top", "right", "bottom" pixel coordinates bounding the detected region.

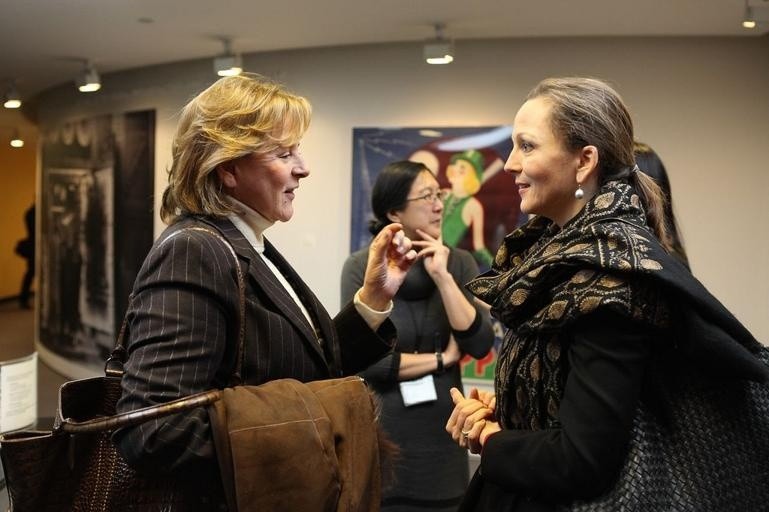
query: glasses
[{"left": 407, "top": 191, "right": 444, "bottom": 203}]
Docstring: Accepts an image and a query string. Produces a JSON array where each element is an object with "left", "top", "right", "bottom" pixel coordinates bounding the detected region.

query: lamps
[
  {"left": 3, "top": 78, "right": 23, "bottom": 109},
  {"left": 74, "top": 59, "right": 101, "bottom": 94},
  {"left": 214, "top": 38, "right": 245, "bottom": 78},
  {"left": 421, "top": 23, "right": 455, "bottom": 66}
]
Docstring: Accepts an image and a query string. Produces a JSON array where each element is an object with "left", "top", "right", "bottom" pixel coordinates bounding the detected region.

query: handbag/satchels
[
  {"left": 0, "top": 371, "right": 248, "bottom": 511},
  {"left": 549, "top": 348, "right": 769, "bottom": 511}
]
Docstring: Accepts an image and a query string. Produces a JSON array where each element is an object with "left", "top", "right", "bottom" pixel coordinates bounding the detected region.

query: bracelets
[{"left": 434, "top": 351, "right": 445, "bottom": 375}]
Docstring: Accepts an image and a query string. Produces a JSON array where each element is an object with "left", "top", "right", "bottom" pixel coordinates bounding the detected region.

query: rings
[{"left": 461, "top": 428, "right": 471, "bottom": 437}]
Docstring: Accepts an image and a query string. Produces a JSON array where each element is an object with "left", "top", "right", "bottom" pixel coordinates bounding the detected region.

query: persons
[
  {"left": 113, "top": 74, "right": 417, "bottom": 510},
  {"left": 445, "top": 75, "right": 678, "bottom": 511},
  {"left": 340, "top": 160, "right": 496, "bottom": 511},
  {"left": 18, "top": 205, "right": 34, "bottom": 310}
]
